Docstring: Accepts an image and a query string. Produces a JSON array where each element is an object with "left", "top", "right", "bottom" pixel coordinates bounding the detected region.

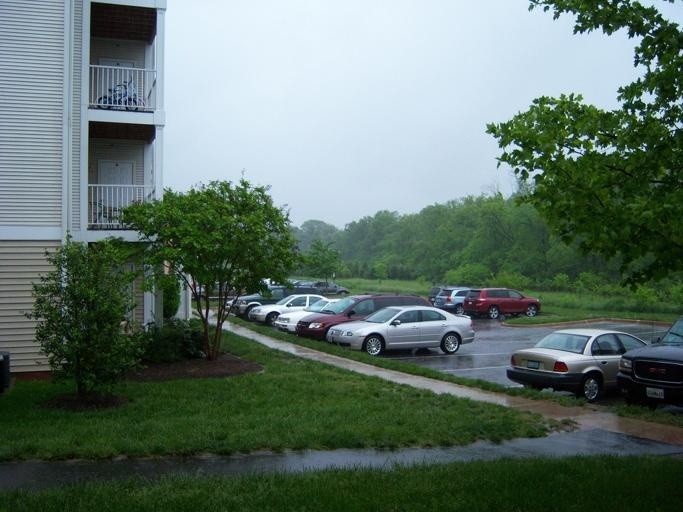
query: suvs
[{"left": 616, "top": 320, "right": 682, "bottom": 409}]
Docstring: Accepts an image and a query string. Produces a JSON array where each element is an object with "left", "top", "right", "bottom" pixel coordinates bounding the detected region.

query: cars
[{"left": 506, "top": 320, "right": 649, "bottom": 405}]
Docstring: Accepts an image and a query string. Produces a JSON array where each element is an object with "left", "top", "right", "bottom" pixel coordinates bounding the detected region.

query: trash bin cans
[{"left": 0, "top": 351, "right": 9, "bottom": 387}]
